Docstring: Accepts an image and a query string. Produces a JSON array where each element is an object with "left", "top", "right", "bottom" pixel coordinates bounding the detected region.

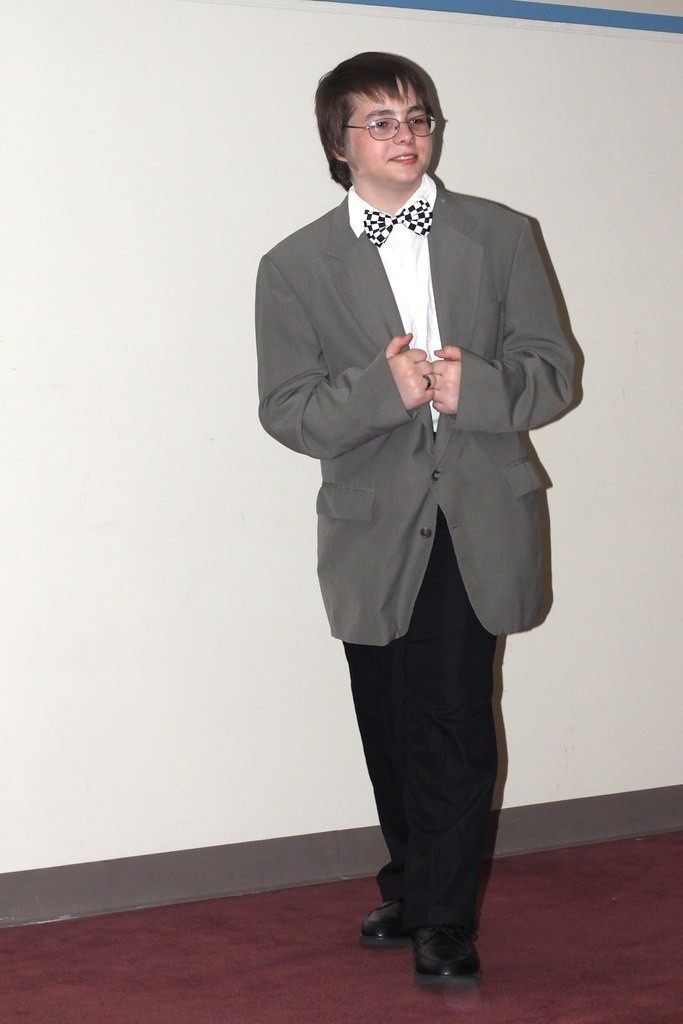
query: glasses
[{"left": 342, "top": 113, "right": 437, "bottom": 140}]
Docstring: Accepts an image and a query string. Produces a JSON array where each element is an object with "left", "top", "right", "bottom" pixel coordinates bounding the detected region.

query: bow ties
[{"left": 364, "top": 197, "right": 433, "bottom": 248}]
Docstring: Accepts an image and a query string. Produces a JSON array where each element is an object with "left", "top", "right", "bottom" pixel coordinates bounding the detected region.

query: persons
[{"left": 255, "top": 52, "right": 583, "bottom": 988}]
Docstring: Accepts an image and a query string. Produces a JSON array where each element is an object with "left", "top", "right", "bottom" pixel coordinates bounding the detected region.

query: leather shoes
[
  {"left": 410, "top": 921, "right": 483, "bottom": 987},
  {"left": 358, "top": 900, "right": 412, "bottom": 949}
]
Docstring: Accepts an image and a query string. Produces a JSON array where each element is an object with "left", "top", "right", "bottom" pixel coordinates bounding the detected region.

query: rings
[{"left": 423, "top": 375, "right": 431, "bottom": 391}]
